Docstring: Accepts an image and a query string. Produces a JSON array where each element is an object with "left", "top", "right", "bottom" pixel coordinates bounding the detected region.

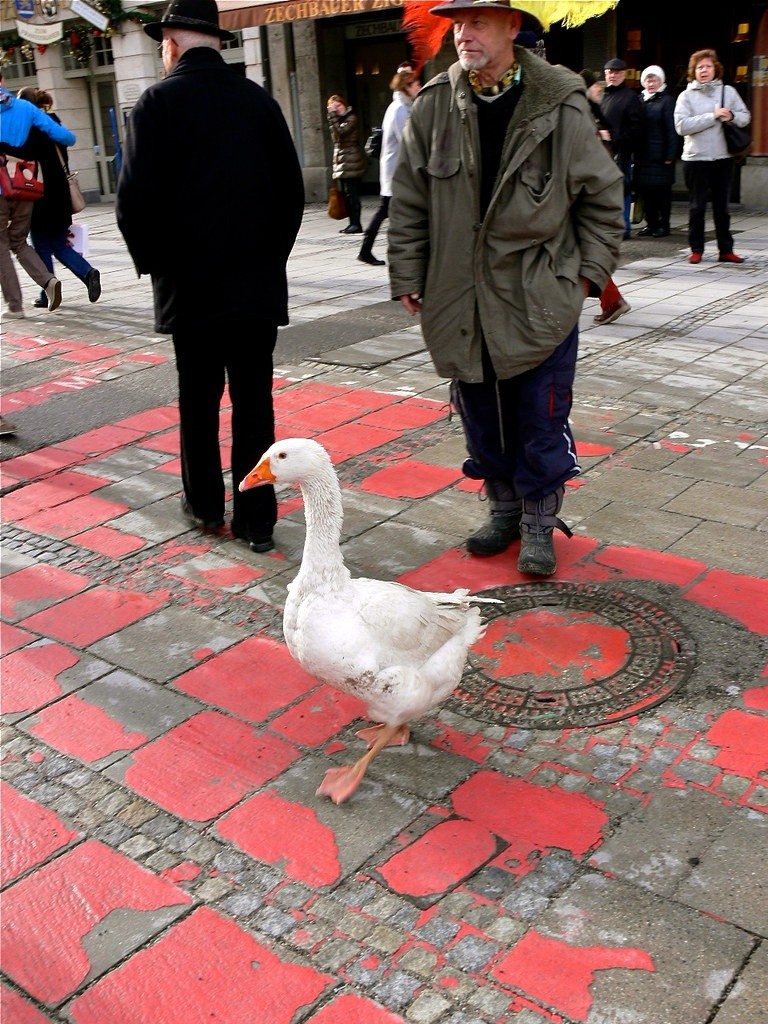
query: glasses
[{"left": 157, "top": 39, "right": 179, "bottom": 52}]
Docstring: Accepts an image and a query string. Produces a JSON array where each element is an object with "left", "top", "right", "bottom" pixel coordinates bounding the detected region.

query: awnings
[{"left": 216, "top": 0, "right": 404, "bottom": 31}]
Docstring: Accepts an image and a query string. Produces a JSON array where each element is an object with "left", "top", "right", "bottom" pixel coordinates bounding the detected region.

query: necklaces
[{"left": 468, "top": 54, "right": 518, "bottom": 96}]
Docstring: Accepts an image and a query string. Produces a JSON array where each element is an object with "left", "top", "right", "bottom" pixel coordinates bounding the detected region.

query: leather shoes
[
  {"left": 592, "top": 299, "right": 631, "bottom": 324},
  {"left": 718, "top": 253, "right": 745, "bottom": 263},
  {"left": 638, "top": 225, "right": 659, "bottom": 235},
  {"left": 652, "top": 228, "right": 671, "bottom": 237},
  {"left": 690, "top": 253, "right": 702, "bottom": 263}
]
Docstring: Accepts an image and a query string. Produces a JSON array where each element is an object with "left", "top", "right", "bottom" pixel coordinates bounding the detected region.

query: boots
[
  {"left": 358, "top": 238, "right": 385, "bottom": 265},
  {"left": 466, "top": 487, "right": 522, "bottom": 555},
  {"left": 517, "top": 483, "right": 574, "bottom": 574}
]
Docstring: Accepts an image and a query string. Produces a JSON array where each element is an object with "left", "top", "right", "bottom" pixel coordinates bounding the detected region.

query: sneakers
[
  {"left": 44, "top": 278, "right": 62, "bottom": 311},
  {"left": 1, "top": 311, "right": 24, "bottom": 318}
]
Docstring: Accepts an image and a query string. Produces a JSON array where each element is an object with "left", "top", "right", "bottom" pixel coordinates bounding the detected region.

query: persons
[
  {"left": 674, "top": 50, "right": 751, "bottom": 264},
  {"left": 397, "top": 62, "right": 414, "bottom": 73},
  {"left": 635, "top": 65, "right": 684, "bottom": 238},
  {"left": 357, "top": 72, "right": 422, "bottom": 265},
  {"left": 387, "top": 0, "right": 626, "bottom": 575},
  {"left": 327, "top": 95, "right": 363, "bottom": 233},
  {"left": 580, "top": 69, "right": 617, "bottom": 152},
  {"left": 0, "top": 73, "right": 76, "bottom": 319},
  {"left": 116, "top": 0, "right": 305, "bottom": 552},
  {"left": 0, "top": 87, "right": 101, "bottom": 308},
  {"left": 599, "top": 59, "right": 652, "bottom": 241}
]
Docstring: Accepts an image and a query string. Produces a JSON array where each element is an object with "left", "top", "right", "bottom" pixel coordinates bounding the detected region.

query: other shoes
[
  {"left": 34, "top": 297, "right": 48, "bottom": 306},
  {"left": 181, "top": 491, "right": 225, "bottom": 528},
  {"left": 231, "top": 519, "right": 274, "bottom": 551},
  {"left": 84, "top": 268, "right": 101, "bottom": 302}
]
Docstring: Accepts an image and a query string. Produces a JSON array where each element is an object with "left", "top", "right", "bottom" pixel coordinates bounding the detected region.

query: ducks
[{"left": 239, "top": 439, "right": 505, "bottom": 805}]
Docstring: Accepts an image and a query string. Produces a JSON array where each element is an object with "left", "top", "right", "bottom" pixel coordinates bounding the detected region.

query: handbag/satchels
[
  {"left": 67, "top": 223, "right": 87, "bottom": 258},
  {"left": 0, "top": 154, "right": 44, "bottom": 201},
  {"left": 67, "top": 171, "right": 85, "bottom": 213},
  {"left": 721, "top": 85, "right": 754, "bottom": 153},
  {"left": 327, "top": 181, "right": 349, "bottom": 219}
]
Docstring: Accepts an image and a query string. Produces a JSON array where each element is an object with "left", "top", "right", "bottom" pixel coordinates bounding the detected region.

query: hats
[
  {"left": 640, "top": 65, "right": 665, "bottom": 88},
  {"left": 389, "top": 65, "right": 418, "bottom": 91},
  {"left": 603, "top": 58, "right": 626, "bottom": 71},
  {"left": 143, "top": 0, "right": 236, "bottom": 41},
  {"left": 580, "top": 68, "right": 597, "bottom": 88},
  {"left": 428, "top": 0, "right": 544, "bottom": 46}
]
